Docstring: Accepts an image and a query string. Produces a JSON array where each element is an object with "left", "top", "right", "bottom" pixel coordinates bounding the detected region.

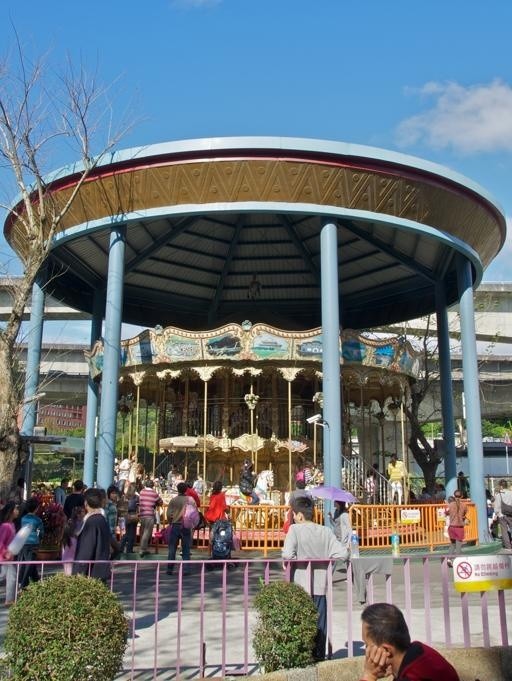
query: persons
[
  {"left": 0, "top": 497, "right": 43, "bottom": 607},
  {"left": 366, "top": 454, "right": 408, "bottom": 505},
  {"left": 295, "top": 460, "right": 322, "bottom": 489},
  {"left": 168, "top": 464, "right": 180, "bottom": 485},
  {"left": 281, "top": 497, "right": 345, "bottom": 663},
  {"left": 361, "top": 603, "right": 459, "bottom": 681},
  {"left": 105, "top": 475, "right": 230, "bottom": 576},
  {"left": 239, "top": 458, "right": 258, "bottom": 504},
  {"left": 446, "top": 472, "right": 470, "bottom": 567},
  {"left": 420, "top": 483, "right": 446, "bottom": 499},
  {"left": 329, "top": 500, "right": 351, "bottom": 547},
  {"left": 54, "top": 479, "right": 111, "bottom": 586},
  {"left": 485, "top": 479, "right": 512, "bottom": 550}
]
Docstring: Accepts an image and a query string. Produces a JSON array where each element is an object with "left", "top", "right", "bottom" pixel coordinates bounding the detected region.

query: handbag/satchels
[
  {"left": 126, "top": 513, "right": 140, "bottom": 525},
  {"left": 184, "top": 498, "right": 200, "bottom": 528}
]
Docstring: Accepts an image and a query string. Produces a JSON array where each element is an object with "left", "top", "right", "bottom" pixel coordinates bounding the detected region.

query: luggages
[{"left": 212, "top": 519, "right": 232, "bottom": 560}]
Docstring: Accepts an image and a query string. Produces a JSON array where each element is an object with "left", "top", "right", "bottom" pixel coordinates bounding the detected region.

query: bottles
[
  {"left": 390, "top": 529, "right": 400, "bottom": 557},
  {"left": 350, "top": 530, "right": 361, "bottom": 560}
]
